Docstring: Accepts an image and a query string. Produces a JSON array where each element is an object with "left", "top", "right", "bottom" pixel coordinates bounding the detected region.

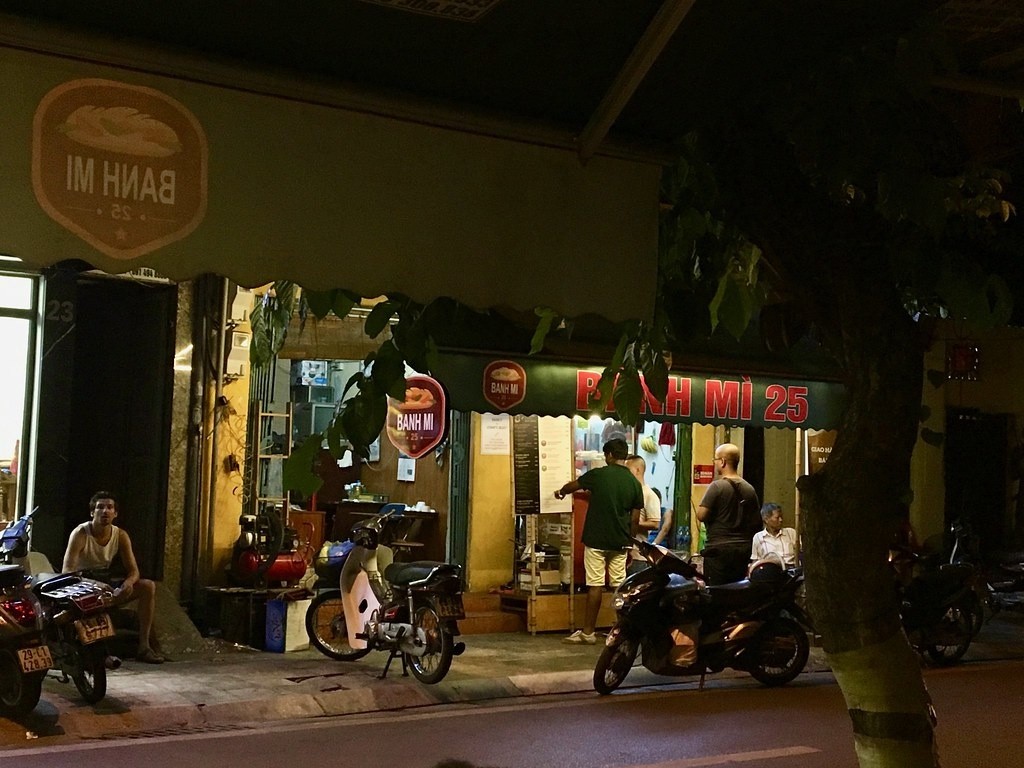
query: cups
[{"left": 352, "top": 485, "right": 361, "bottom": 499}]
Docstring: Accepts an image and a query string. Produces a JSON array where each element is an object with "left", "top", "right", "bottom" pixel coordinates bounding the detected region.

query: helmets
[{"left": 748, "top": 560, "right": 782, "bottom": 583}]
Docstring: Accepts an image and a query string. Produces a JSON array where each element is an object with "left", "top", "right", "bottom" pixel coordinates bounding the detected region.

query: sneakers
[
  {"left": 136, "top": 646, "right": 165, "bottom": 664},
  {"left": 562, "top": 630, "right": 597, "bottom": 645}
]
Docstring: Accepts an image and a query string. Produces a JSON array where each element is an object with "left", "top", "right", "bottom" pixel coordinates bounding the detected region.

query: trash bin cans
[{"left": 200, "top": 584, "right": 310, "bottom": 653}]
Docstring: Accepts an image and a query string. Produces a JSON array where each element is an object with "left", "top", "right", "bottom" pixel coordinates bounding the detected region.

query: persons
[
  {"left": 749, "top": 502, "right": 803, "bottom": 602},
  {"left": 697, "top": 443, "right": 764, "bottom": 586},
  {"left": 61, "top": 491, "right": 164, "bottom": 670},
  {"left": 553, "top": 439, "right": 645, "bottom": 644},
  {"left": 624, "top": 455, "right": 673, "bottom": 578},
  {"left": 888, "top": 503, "right": 916, "bottom": 586}
]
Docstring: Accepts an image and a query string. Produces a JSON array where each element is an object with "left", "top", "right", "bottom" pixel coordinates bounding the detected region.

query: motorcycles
[
  {"left": 304, "top": 509, "right": 468, "bottom": 684},
  {"left": 887, "top": 523, "right": 1024, "bottom": 668},
  {"left": 593, "top": 529, "right": 813, "bottom": 696},
  {"left": 0, "top": 505, "right": 122, "bottom": 719}
]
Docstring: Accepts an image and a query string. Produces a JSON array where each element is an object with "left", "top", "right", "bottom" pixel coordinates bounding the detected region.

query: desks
[
  {"left": 208, "top": 585, "right": 300, "bottom": 647},
  {"left": 322, "top": 501, "right": 387, "bottom": 543},
  {"left": 353, "top": 512, "right": 439, "bottom": 563}
]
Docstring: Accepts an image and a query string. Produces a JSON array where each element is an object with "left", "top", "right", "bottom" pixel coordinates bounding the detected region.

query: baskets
[{"left": 313, "top": 544, "right": 348, "bottom": 577}]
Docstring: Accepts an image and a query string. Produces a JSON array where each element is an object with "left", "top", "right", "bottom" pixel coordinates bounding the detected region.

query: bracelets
[{"left": 558, "top": 490, "right": 565, "bottom": 498}]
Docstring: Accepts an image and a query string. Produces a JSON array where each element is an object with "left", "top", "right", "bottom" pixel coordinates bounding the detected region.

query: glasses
[{"left": 711, "top": 456, "right": 722, "bottom": 464}]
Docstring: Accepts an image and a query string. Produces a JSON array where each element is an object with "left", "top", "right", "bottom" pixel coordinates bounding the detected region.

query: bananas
[{"left": 640, "top": 436, "right": 657, "bottom": 453}]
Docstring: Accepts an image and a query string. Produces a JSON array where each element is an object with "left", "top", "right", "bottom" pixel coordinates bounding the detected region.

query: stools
[{"left": 390, "top": 542, "right": 424, "bottom": 561}]
